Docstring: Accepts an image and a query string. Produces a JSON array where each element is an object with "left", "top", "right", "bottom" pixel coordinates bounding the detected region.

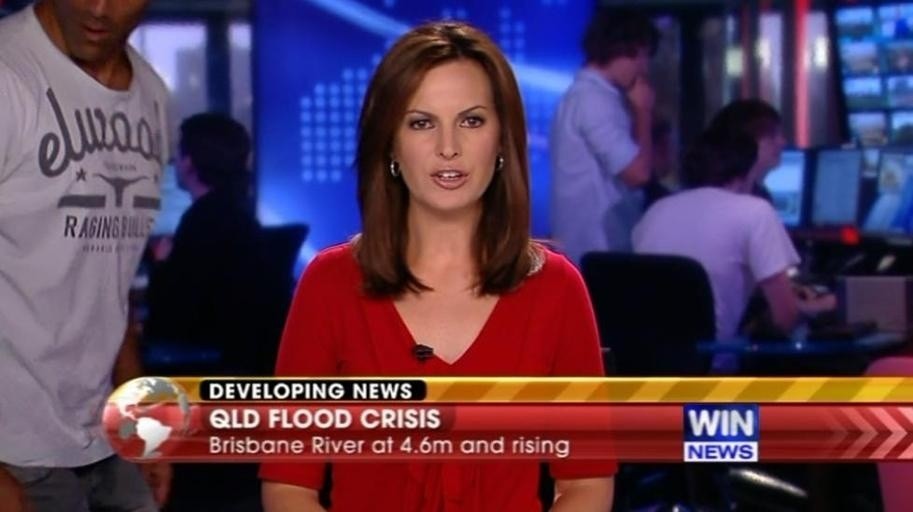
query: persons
[
  {"left": 549, "top": 2, "right": 673, "bottom": 275},
  {"left": 624, "top": 127, "right": 887, "bottom": 512},
  {"left": 0, "top": 2, "right": 177, "bottom": 511},
  {"left": 256, "top": 20, "right": 622, "bottom": 511},
  {"left": 723, "top": 94, "right": 838, "bottom": 317},
  {"left": 136, "top": 108, "right": 282, "bottom": 358}
]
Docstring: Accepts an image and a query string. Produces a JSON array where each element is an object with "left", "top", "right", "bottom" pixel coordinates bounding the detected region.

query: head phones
[{"left": 722, "top": 129, "right": 758, "bottom": 169}]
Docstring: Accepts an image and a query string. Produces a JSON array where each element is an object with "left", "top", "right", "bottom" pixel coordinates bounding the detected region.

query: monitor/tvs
[
  {"left": 825, "top": 1, "right": 912, "bottom": 146},
  {"left": 861, "top": 146, "right": 913, "bottom": 237},
  {"left": 763, "top": 149, "right": 813, "bottom": 227},
  {"left": 808, "top": 143, "right": 864, "bottom": 226}
]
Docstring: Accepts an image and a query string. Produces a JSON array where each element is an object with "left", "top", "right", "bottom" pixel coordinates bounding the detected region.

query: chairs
[
  {"left": 579, "top": 249, "right": 817, "bottom": 512},
  {"left": 138, "top": 220, "right": 311, "bottom": 377},
  {"left": 862, "top": 357, "right": 913, "bottom": 512}
]
